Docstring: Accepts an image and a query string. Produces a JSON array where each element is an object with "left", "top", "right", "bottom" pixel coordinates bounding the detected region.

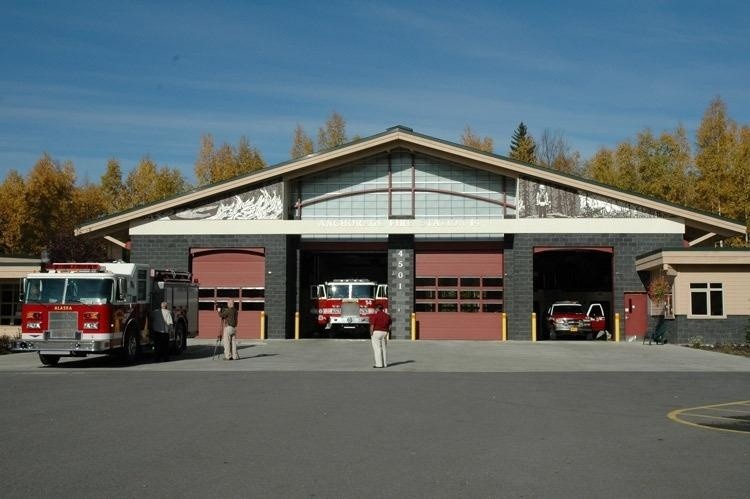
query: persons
[
  {"left": 216, "top": 298, "right": 238, "bottom": 361},
  {"left": 369, "top": 305, "right": 392, "bottom": 369},
  {"left": 150, "top": 302, "right": 176, "bottom": 362}
]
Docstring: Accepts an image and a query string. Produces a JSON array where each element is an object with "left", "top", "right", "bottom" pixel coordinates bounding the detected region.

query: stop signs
[
  {"left": 307, "top": 277, "right": 389, "bottom": 339},
  {"left": 18, "top": 258, "right": 203, "bottom": 366}
]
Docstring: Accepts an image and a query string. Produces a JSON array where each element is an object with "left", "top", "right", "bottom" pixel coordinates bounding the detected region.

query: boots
[{"left": 547, "top": 298, "right": 608, "bottom": 341}]
